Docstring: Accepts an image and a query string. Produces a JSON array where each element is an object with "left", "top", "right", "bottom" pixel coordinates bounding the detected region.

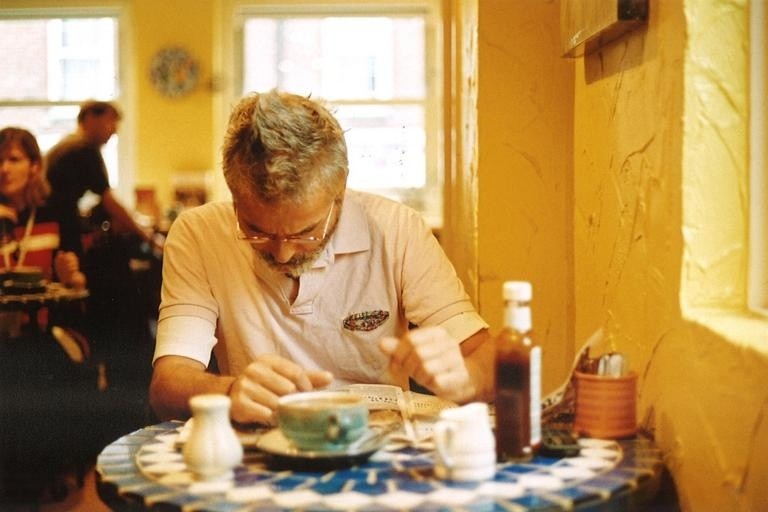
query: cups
[
  {"left": 275, "top": 389, "right": 372, "bottom": 453},
  {"left": 429, "top": 399, "right": 499, "bottom": 485}
]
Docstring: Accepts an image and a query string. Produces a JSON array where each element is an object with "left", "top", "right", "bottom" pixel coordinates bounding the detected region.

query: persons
[
  {"left": 39, "top": 99, "right": 163, "bottom": 396},
  {"left": 1, "top": 125, "right": 109, "bottom": 505},
  {"left": 148, "top": 89, "right": 499, "bottom": 427}
]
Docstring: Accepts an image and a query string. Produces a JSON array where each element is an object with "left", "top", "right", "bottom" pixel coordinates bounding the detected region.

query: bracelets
[{"left": 224, "top": 375, "right": 241, "bottom": 397}]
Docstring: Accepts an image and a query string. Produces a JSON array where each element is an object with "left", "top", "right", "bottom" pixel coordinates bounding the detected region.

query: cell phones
[{"left": 541, "top": 429, "right": 579, "bottom": 457}]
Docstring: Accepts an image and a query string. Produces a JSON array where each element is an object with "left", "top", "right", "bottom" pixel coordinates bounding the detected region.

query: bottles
[
  {"left": 493, "top": 278, "right": 543, "bottom": 465},
  {"left": 183, "top": 392, "right": 246, "bottom": 480}
]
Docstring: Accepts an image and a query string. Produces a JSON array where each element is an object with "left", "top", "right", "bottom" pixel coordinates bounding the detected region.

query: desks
[
  {"left": 1, "top": 275, "right": 92, "bottom": 508},
  {"left": 92, "top": 390, "right": 669, "bottom": 510}
]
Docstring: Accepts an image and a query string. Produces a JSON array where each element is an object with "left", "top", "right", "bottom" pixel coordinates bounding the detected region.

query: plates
[{"left": 254, "top": 424, "right": 391, "bottom": 459}]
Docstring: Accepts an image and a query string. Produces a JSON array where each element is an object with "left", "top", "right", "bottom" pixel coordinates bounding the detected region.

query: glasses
[{"left": 237, "top": 199, "right": 335, "bottom": 244}]
